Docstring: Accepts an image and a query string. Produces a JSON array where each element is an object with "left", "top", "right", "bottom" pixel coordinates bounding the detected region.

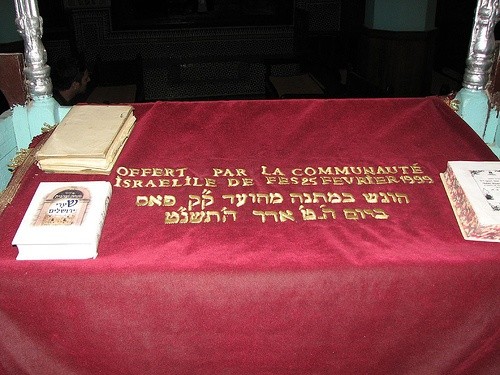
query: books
[
  {"left": 35, "top": 103, "right": 136, "bottom": 178},
  {"left": 11, "top": 181, "right": 112, "bottom": 259},
  {"left": 440, "top": 161, "right": 500, "bottom": 243}
]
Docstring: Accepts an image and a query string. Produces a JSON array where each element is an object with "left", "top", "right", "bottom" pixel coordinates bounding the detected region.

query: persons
[{"left": 51, "top": 58, "right": 92, "bottom": 105}]
